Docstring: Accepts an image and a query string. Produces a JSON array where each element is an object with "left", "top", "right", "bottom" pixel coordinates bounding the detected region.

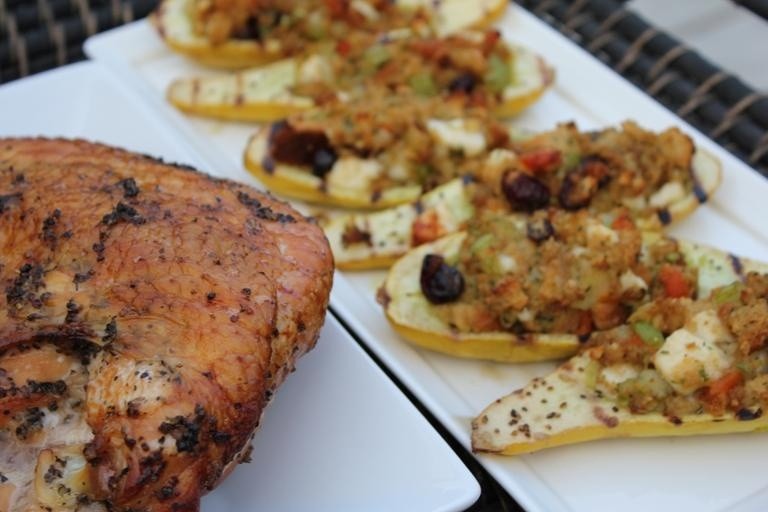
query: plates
[
  {"left": 0, "top": 59, "right": 486, "bottom": 511},
  {"left": 81, "top": 0, "right": 768, "bottom": 512}
]
